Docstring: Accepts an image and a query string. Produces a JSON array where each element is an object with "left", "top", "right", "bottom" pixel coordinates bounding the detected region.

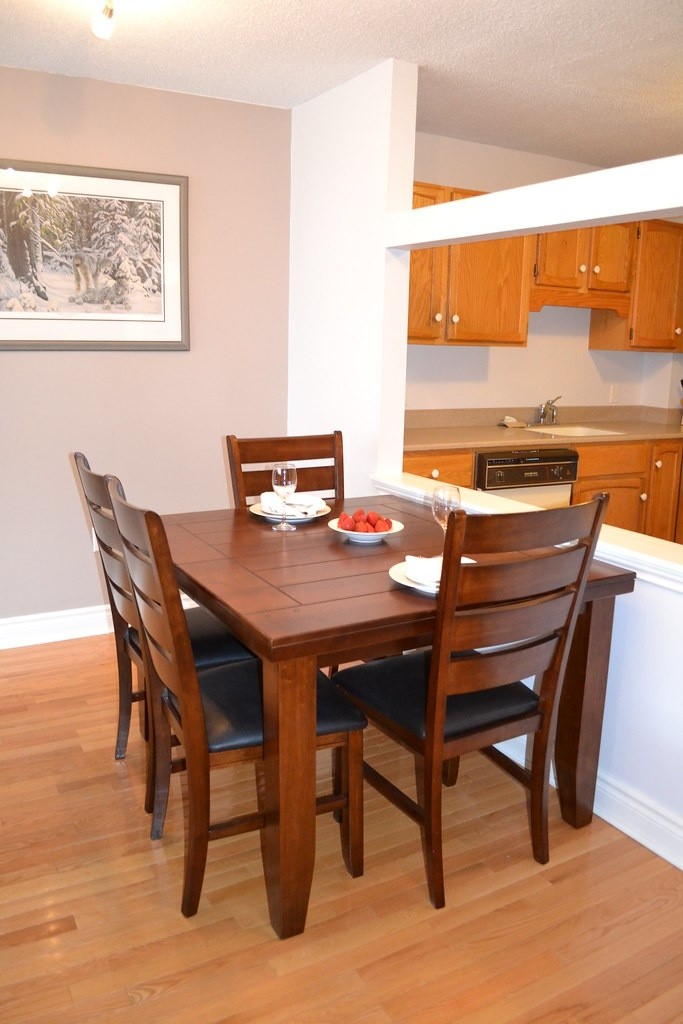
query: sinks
[{"left": 524, "top": 426, "right": 626, "bottom": 436}]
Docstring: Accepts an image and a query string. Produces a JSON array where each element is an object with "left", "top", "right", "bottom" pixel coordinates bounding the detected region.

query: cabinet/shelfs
[
  {"left": 402, "top": 452, "right": 478, "bottom": 489},
  {"left": 566, "top": 444, "right": 656, "bottom": 536},
  {"left": 530, "top": 217, "right": 639, "bottom": 294},
  {"left": 590, "top": 220, "right": 683, "bottom": 352},
  {"left": 408, "top": 180, "right": 528, "bottom": 348},
  {"left": 644, "top": 442, "right": 683, "bottom": 544}
]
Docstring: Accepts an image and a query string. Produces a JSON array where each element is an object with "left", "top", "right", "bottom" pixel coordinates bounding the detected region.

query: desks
[{"left": 140, "top": 493, "right": 636, "bottom": 942}]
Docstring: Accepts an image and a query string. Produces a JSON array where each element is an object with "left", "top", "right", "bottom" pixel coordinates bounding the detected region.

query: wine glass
[
  {"left": 272, "top": 463, "right": 298, "bottom": 531},
  {"left": 432, "top": 485, "right": 461, "bottom": 558}
]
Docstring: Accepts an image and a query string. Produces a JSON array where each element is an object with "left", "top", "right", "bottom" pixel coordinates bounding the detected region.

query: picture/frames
[{"left": 0, "top": 159, "right": 191, "bottom": 353}]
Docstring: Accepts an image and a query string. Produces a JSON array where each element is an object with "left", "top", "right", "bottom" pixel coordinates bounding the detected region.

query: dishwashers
[{"left": 476, "top": 450, "right": 578, "bottom": 509}]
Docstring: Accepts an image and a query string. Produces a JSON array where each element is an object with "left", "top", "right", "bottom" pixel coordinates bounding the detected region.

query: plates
[
  {"left": 249, "top": 503, "right": 332, "bottom": 521},
  {"left": 388, "top": 558, "right": 444, "bottom": 596}
]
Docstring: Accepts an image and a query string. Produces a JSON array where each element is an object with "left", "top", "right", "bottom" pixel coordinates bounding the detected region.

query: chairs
[
  {"left": 226, "top": 432, "right": 339, "bottom": 679},
  {"left": 106, "top": 478, "right": 369, "bottom": 918},
  {"left": 334, "top": 492, "right": 610, "bottom": 909},
  {"left": 72, "top": 452, "right": 260, "bottom": 814}
]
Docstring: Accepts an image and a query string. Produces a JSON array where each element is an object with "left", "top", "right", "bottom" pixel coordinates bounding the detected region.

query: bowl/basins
[{"left": 328, "top": 516, "right": 404, "bottom": 543}]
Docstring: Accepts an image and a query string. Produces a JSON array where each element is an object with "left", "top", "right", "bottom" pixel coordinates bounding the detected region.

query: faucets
[{"left": 535, "top": 395, "right": 563, "bottom": 425}]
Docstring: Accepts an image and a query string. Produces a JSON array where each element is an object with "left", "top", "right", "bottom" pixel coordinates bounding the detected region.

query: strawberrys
[{"left": 338, "top": 509, "right": 392, "bottom": 532}]
